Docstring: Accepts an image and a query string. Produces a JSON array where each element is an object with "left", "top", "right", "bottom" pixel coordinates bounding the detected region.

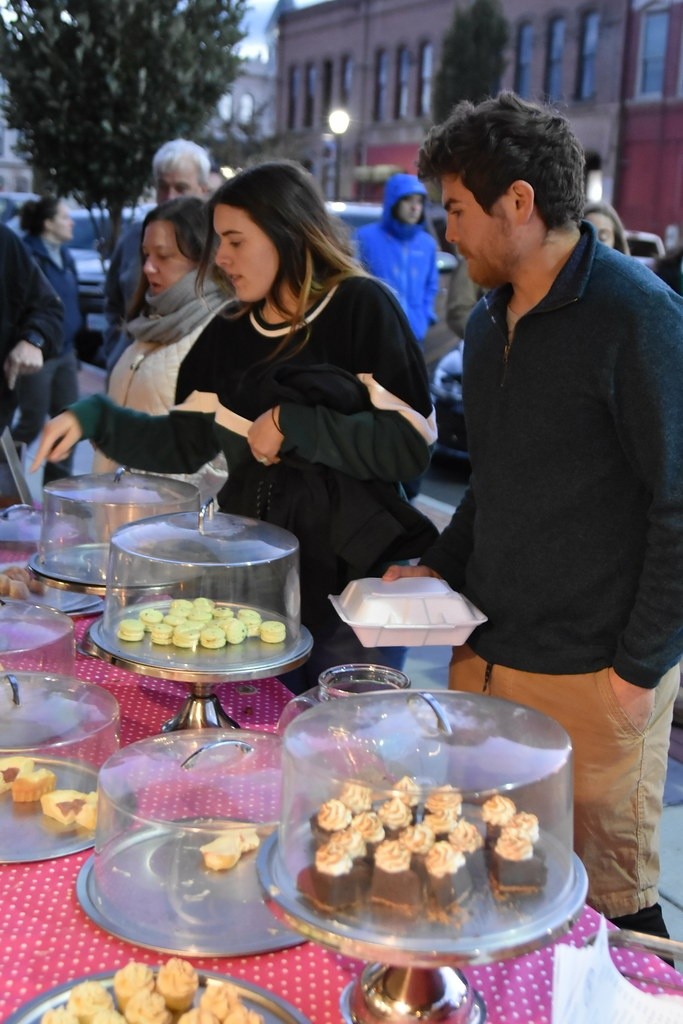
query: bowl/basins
[{"left": 327, "top": 577, "right": 488, "bottom": 648}]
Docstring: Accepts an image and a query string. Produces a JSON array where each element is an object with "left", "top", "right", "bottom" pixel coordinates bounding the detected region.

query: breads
[
  {"left": 199, "top": 823, "right": 260, "bottom": 870},
  {"left": 1, "top": 756, "right": 108, "bottom": 830},
  {"left": 0, "top": 566, "right": 48, "bottom": 600}
]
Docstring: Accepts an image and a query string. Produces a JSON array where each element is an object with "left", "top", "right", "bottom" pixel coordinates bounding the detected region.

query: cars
[
  {"left": 427, "top": 341, "right": 472, "bottom": 462},
  {"left": 0, "top": 188, "right": 462, "bottom": 404}
]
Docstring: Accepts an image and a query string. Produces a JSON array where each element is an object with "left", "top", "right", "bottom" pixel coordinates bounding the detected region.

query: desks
[{"left": 0, "top": 501, "right": 683, "bottom": 1024}]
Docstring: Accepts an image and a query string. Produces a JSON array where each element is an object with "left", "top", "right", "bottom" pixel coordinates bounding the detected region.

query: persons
[
  {"left": 577, "top": 200, "right": 629, "bottom": 261},
  {"left": 0, "top": 218, "right": 65, "bottom": 435},
  {"left": 92, "top": 200, "right": 228, "bottom": 497},
  {"left": 18, "top": 196, "right": 82, "bottom": 442},
  {"left": 350, "top": 173, "right": 436, "bottom": 347},
  {"left": 28, "top": 163, "right": 437, "bottom": 692},
  {"left": 102, "top": 137, "right": 210, "bottom": 392},
  {"left": 383, "top": 90, "right": 683, "bottom": 963}
]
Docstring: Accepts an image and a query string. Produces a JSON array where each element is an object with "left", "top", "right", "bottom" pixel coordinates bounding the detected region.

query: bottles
[{"left": 275, "top": 663, "right": 411, "bottom": 741}]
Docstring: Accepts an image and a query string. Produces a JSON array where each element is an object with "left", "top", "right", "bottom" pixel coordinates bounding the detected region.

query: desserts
[
  {"left": 296, "top": 775, "right": 547, "bottom": 914},
  {"left": 116, "top": 597, "right": 289, "bottom": 648},
  {"left": 38, "top": 959, "right": 265, "bottom": 1024}
]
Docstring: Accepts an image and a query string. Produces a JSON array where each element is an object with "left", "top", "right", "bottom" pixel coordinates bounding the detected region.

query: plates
[{"left": 0, "top": 560, "right": 109, "bottom": 621}]
